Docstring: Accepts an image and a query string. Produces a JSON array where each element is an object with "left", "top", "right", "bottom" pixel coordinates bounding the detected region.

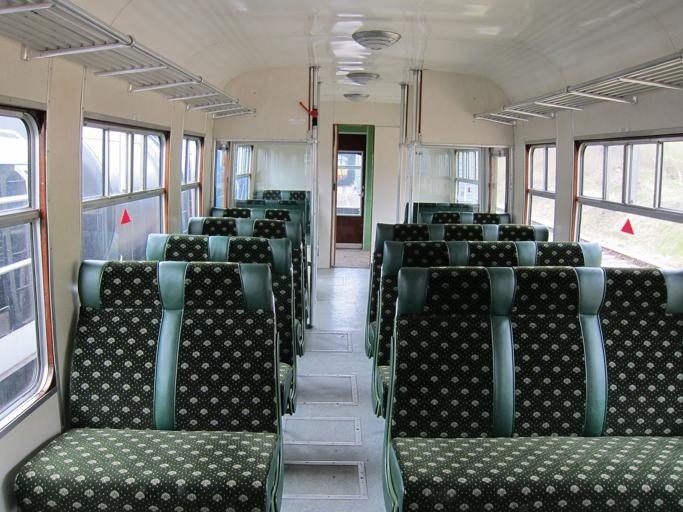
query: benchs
[
  {"left": 372, "top": 241, "right": 602, "bottom": 419},
  {"left": 234, "top": 200, "right": 306, "bottom": 227},
  {"left": 417, "top": 212, "right": 511, "bottom": 224},
  {"left": 364, "top": 223, "right": 549, "bottom": 359},
  {"left": 253, "top": 190, "right": 310, "bottom": 233},
  {"left": 210, "top": 207, "right": 309, "bottom": 318},
  {"left": 146, "top": 234, "right": 296, "bottom": 416},
  {"left": 404, "top": 202, "right": 474, "bottom": 224},
  {"left": 382, "top": 267, "right": 683, "bottom": 512},
  {"left": 189, "top": 217, "right": 305, "bottom": 358},
  {"left": 13, "top": 260, "right": 283, "bottom": 512}
]
